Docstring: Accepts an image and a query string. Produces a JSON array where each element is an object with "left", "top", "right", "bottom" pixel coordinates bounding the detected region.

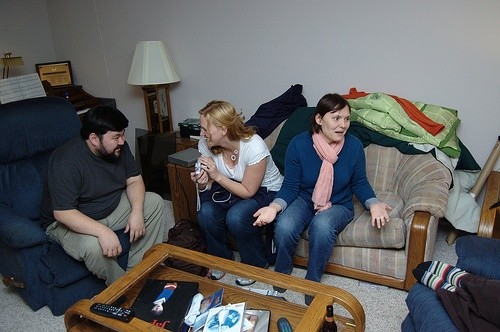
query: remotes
[
  {"left": 277, "top": 317, "right": 292, "bottom": 332},
  {"left": 90, "top": 303, "right": 136, "bottom": 322}
]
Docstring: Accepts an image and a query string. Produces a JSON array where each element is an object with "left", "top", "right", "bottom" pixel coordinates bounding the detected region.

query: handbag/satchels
[{"left": 162, "top": 218, "right": 210, "bottom": 278}]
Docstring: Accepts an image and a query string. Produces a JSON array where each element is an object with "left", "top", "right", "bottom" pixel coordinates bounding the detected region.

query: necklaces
[{"left": 222, "top": 140, "right": 238, "bottom": 162}]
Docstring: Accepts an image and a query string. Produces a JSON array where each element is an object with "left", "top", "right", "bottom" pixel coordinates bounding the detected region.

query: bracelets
[{"left": 198, "top": 185, "right": 209, "bottom": 193}]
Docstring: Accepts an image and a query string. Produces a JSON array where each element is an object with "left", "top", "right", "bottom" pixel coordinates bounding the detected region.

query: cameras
[{"left": 195, "top": 157, "right": 203, "bottom": 176}]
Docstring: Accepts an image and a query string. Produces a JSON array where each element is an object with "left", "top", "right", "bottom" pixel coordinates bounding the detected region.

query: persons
[
  {"left": 138, "top": 280, "right": 193, "bottom": 319},
  {"left": 40, "top": 105, "right": 163, "bottom": 294},
  {"left": 192, "top": 101, "right": 287, "bottom": 285},
  {"left": 411, "top": 260, "right": 500, "bottom": 332},
  {"left": 252, "top": 92, "right": 394, "bottom": 310}
]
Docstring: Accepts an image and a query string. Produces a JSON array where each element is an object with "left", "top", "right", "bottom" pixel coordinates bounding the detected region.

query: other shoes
[
  {"left": 235, "top": 261, "right": 269, "bottom": 286},
  {"left": 207, "top": 268, "right": 226, "bottom": 279}
]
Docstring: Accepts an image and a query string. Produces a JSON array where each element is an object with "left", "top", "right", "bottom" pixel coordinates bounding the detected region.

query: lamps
[
  {"left": 127, "top": 41, "right": 180, "bottom": 133},
  {"left": 0, "top": 52, "right": 25, "bottom": 79}
]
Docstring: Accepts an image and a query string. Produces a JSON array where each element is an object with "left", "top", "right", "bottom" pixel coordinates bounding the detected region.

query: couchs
[
  {"left": 0, "top": 96, "right": 166, "bottom": 316},
  {"left": 400, "top": 235, "right": 500, "bottom": 332},
  {"left": 168, "top": 143, "right": 453, "bottom": 292}
]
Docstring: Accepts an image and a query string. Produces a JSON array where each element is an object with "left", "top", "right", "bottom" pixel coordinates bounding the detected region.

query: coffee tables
[{"left": 64, "top": 243, "right": 365, "bottom": 332}]
